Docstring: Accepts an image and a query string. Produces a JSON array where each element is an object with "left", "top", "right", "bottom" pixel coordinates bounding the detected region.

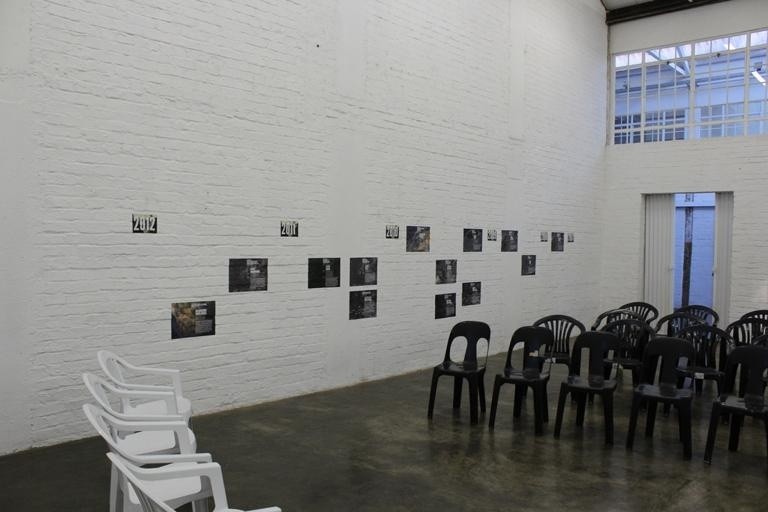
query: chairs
[
  {"left": 554, "top": 330, "right": 625, "bottom": 452},
  {"left": 82, "top": 349, "right": 285, "bottom": 512},
  {"left": 489, "top": 324, "right": 555, "bottom": 437},
  {"left": 532, "top": 302, "right": 767, "bottom": 426},
  {"left": 428, "top": 320, "right": 492, "bottom": 428},
  {"left": 626, "top": 335, "right": 696, "bottom": 461},
  {"left": 703, "top": 345, "right": 767, "bottom": 464}
]
